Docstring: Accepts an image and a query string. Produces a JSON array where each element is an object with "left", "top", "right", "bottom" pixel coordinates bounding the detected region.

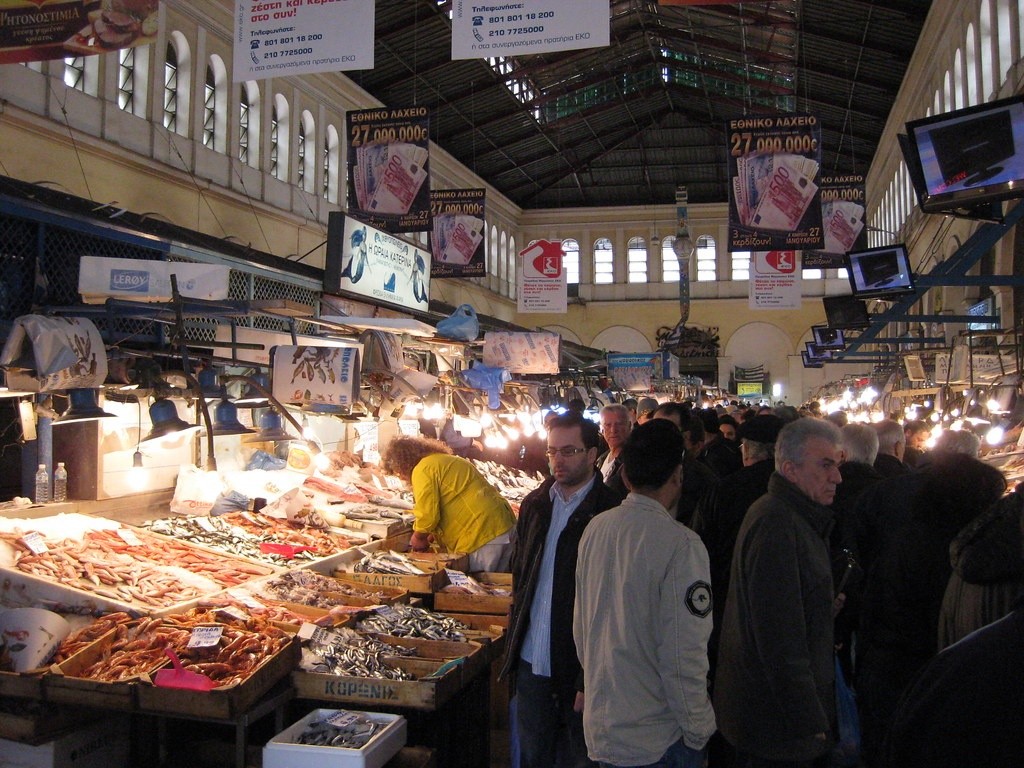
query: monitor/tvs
[
  {"left": 800, "top": 242, "right": 914, "bottom": 367},
  {"left": 897, "top": 95, "right": 1024, "bottom": 221}
]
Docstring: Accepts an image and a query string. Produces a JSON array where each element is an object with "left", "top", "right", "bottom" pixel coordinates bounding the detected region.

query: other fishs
[{"left": 144, "top": 458, "right": 546, "bottom": 752}]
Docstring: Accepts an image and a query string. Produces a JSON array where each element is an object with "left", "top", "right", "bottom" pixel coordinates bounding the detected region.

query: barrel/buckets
[{"left": 286, "top": 434, "right": 323, "bottom": 476}]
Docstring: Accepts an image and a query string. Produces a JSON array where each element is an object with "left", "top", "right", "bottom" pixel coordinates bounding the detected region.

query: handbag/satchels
[
  {"left": 436, "top": 303, "right": 480, "bottom": 342},
  {"left": 832, "top": 653, "right": 862, "bottom": 768},
  {"left": 510, "top": 691, "right": 522, "bottom": 767}
]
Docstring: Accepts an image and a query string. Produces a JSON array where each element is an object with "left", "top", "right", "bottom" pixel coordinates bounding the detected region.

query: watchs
[{"left": 671, "top": 185, "right": 696, "bottom": 321}]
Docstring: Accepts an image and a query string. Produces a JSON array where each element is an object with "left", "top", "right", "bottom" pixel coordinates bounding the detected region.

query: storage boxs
[{"left": 0, "top": 446, "right": 550, "bottom": 768}]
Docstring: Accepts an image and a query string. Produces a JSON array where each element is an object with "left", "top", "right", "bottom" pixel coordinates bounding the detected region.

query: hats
[
  {"left": 624, "top": 417, "right": 686, "bottom": 473},
  {"left": 636, "top": 397, "right": 658, "bottom": 421},
  {"left": 737, "top": 414, "right": 786, "bottom": 442},
  {"left": 712, "top": 407, "right": 727, "bottom": 414},
  {"left": 726, "top": 405, "right": 738, "bottom": 413}
]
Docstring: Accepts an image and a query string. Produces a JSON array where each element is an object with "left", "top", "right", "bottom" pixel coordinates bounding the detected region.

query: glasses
[{"left": 546, "top": 447, "right": 587, "bottom": 457}]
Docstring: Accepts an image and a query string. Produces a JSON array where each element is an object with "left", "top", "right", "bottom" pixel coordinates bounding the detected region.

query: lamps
[
  {"left": 243, "top": 410, "right": 297, "bottom": 444},
  {"left": 136, "top": 399, "right": 205, "bottom": 447},
  {"left": 197, "top": 401, "right": 256, "bottom": 438},
  {"left": 49, "top": 388, "right": 117, "bottom": 424},
  {"left": 120, "top": 360, "right": 174, "bottom": 391},
  {"left": 198, "top": 368, "right": 220, "bottom": 392},
  {"left": 234, "top": 372, "right": 271, "bottom": 401}
]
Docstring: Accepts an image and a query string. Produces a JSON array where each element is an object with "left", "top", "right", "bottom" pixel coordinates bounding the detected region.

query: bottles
[
  {"left": 36, "top": 464, "right": 48, "bottom": 504},
  {"left": 53, "top": 462, "right": 68, "bottom": 502}
]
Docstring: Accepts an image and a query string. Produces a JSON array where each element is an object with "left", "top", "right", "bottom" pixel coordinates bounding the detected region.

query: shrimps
[{"left": 0, "top": 522, "right": 336, "bottom": 689}]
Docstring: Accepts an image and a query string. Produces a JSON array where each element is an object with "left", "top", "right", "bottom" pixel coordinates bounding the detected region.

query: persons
[
  {"left": 383, "top": 436, "right": 518, "bottom": 572},
  {"left": 573, "top": 419, "right": 719, "bottom": 768},
  {"left": 708, "top": 416, "right": 843, "bottom": 768},
  {"left": 414, "top": 414, "right": 437, "bottom": 439},
  {"left": 498, "top": 397, "right": 1024, "bottom": 768},
  {"left": 441, "top": 416, "right": 484, "bottom": 459}
]
